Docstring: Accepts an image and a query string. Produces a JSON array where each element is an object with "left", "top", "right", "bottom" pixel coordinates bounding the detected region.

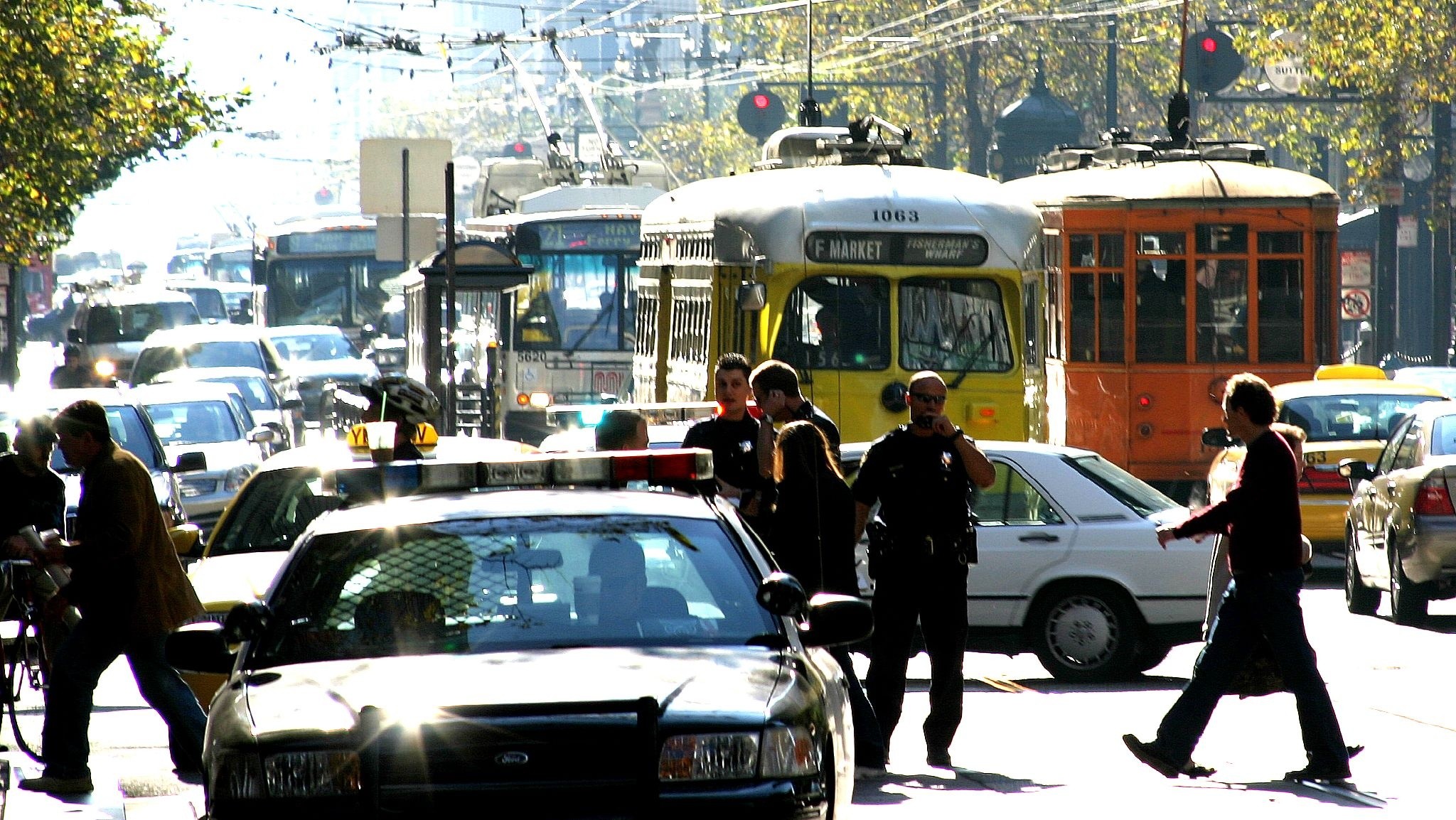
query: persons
[
  {"left": 850, "top": 368, "right": 995, "bottom": 776},
  {"left": 17, "top": 399, "right": 211, "bottom": 796},
  {"left": 1166, "top": 424, "right": 1366, "bottom": 777},
  {"left": 15, "top": 257, "right": 441, "bottom": 499},
  {"left": 1067, "top": 249, "right": 1408, "bottom": 435},
  {"left": 763, "top": 420, "right": 891, "bottom": 781},
  {"left": 535, "top": 238, "right": 872, "bottom": 362},
  {"left": 1124, "top": 371, "right": 1352, "bottom": 782},
  {"left": 679, "top": 352, "right": 780, "bottom": 553},
  {"left": 713, "top": 360, "right": 841, "bottom": 541},
  {"left": 1, "top": 414, "right": 67, "bottom": 710},
  {"left": 548, "top": 536, "right": 671, "bottom": 642},
  {"left": 594, "top": 409, "right": 650, "bottom": 452}
]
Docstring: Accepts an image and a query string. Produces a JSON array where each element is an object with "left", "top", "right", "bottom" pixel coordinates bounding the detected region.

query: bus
[
  {"left": 455, "top": 27, "right": 665, "bottom": 445},
  {"left": 630, "top": 94, "right": 1048, "bottom": 520},
  {"left": 999, "top": 94, "right": 1342, "bottom": 502}
]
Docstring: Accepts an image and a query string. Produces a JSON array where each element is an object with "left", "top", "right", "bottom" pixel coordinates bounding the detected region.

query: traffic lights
[
  {"left": 748, "top": 87, "right": 777, "bottom": 142},
  {"left": 1194, "top": 31, "right": 1221, "bottom": 92}
]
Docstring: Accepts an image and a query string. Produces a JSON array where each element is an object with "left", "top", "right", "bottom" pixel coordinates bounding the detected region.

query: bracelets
[{"left": 948, "top": 429, "right": 964, "bottom": 441}]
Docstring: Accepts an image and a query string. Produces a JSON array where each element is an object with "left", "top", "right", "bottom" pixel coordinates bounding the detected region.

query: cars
[
  {"left": 165, "top": 420, "right": 552, "bottom": 724},
  {"left": 170, "top": 449, "right": 875, "bottom": 820},
  {"left": 0, "top": 208, "right": 410, "bottom": 560},
  {"left": 838, "top": 440, "right": 1318, "bottom": 687},
  {"left": 1199, "top": 364, "right": 1451, "bottom": 544},
  {"left": 1335, "top": 401, "right": 1456, "bottom": 623}
]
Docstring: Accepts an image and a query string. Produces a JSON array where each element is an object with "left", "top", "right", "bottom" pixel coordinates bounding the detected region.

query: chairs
[{"left": 353, "top": 590, "right": 446, "bottom": 645}]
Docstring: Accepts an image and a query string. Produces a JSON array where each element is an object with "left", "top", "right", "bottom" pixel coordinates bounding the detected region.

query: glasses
[
  {"left": 909, "top": 391, "right": 946, "bottom": 405},
  {"left": 754, "top": 389, "right": 770, "bottom": 405}
]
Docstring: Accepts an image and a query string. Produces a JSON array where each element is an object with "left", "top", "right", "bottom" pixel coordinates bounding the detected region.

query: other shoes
[
  {"left": 855, "top": 763, "right": 888, "bottom": 781},
  {"left": 17, "top": 768, "right": 94, "bottom": 793},
  {"left": 1283, "top": 763, "right": 1352, "bottom": 781},
  {"left": 1123, "top": 733, "right": 1179, "bottom": 778}
]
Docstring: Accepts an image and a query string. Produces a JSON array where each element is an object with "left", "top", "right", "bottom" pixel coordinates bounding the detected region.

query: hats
[
  {"left": 52, "top": 400, "right": 112, "bottom": 440},
  {"left": 62, "top": 345, "right": 80, "bottom": 357},
  {"left": 16, "top": 416, "right": 57, "bottom": 442}
]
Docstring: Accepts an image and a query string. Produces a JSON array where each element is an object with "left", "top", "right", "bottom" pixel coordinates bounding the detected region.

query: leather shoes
[{"left": 926, "top": 742, "right": 951, "bottom": 765}]
